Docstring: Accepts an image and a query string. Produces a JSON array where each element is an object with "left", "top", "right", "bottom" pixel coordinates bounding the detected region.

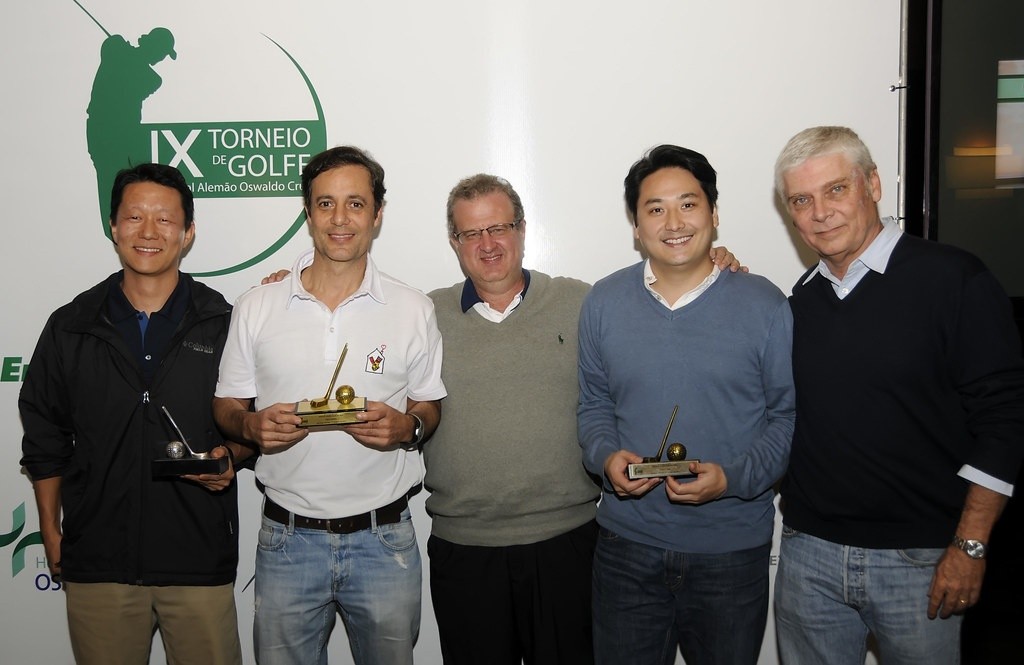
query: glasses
[{"left": 454, "top": 220, "right": 521, "bottom": 246}]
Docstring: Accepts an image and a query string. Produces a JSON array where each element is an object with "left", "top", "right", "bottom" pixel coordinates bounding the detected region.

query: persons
[
  {"left": 214, "top": 143, "right": 452, "bottom": 665},
  {"left": 705, "top": 125, "right": 1024, "bottom": 663},
  {"left": 578, "top": 144, "right": 799, "bottom": 665},
  {"left": 14, "top": 163, "right": 255, "bottom": 665},
  {"left": 261, "top": 171, "right": 753, "bottom": 665}
]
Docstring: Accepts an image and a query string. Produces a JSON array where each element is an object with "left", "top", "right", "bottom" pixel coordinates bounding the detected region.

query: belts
[{"left": 264, "top": 495, "right": 408, "bottom": 535}]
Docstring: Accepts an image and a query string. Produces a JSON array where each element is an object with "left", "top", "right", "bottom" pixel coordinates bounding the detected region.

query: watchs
[
  {"left": 951, "top": 534, "right": 987, "bottom": 558},
  {"left": 403, "top": 413, "right": 426, "bottom": 448}
]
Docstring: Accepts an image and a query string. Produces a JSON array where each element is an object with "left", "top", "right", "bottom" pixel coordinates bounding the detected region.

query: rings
[{"left": 958, "top": 597, "right": 968, "bottom": 606}]
